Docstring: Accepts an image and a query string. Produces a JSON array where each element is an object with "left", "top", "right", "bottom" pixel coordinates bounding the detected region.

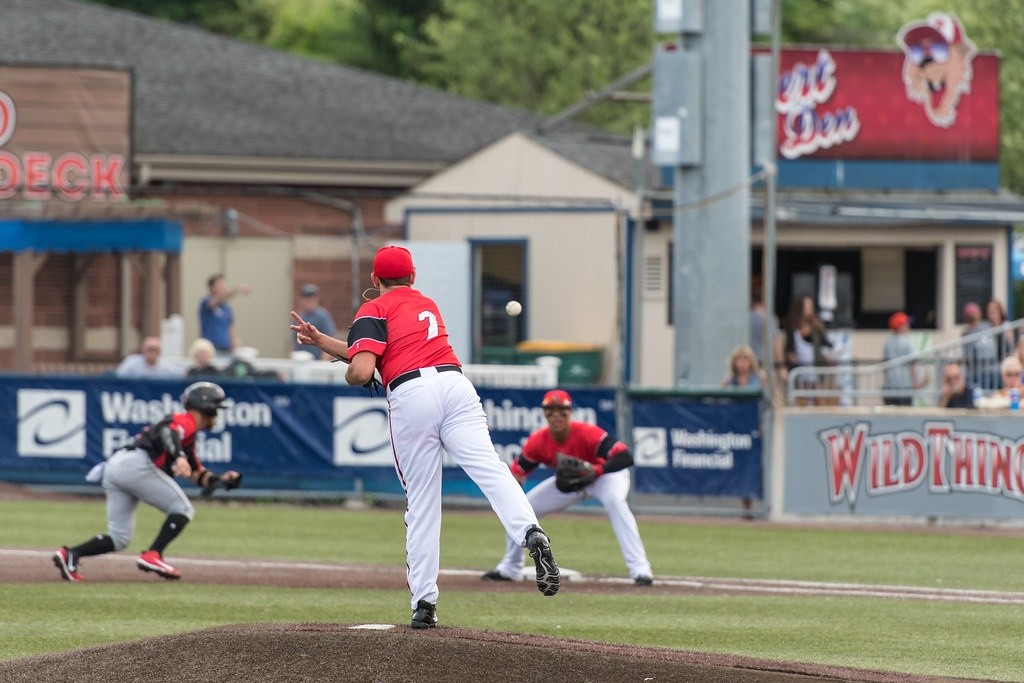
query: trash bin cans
[{"left": 517, "top": 340, "right": 605, "bottom": 386}]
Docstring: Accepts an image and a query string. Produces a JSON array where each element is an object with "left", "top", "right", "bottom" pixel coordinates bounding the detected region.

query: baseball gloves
[
  {"left": 201, "top": 469, "right": 244, "bottom": 498},
  {"left": 556, "top": 452, "right": 596, "bottom": 494}
]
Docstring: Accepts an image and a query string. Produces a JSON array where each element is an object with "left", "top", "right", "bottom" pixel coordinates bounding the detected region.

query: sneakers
[
  {"left": 136, "top": 550, "right": 181, "bottom": 579},
  {"left": 526, "top": 528, "right": 561, "bottom": 597},
  {"left": 53, "top": 545, "right": 85, "bottom": 582},
  {"left": 411, "top": 600, "right": 439, "bottom": 629}
]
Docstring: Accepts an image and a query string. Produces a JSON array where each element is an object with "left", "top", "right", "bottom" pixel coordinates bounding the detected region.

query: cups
[{"left": 1008, "top": 388, "right": 1022, "bottom": 410}]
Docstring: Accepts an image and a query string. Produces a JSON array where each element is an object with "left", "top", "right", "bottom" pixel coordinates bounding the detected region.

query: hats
[
  {"left": 542, "top": 391, "right": 572, "bottom": 409},
  {"left": 373, "top": 246, "right": 416, "bottom": 278},
  {"left": 302, "top": 284, "right": 318, "bottom": 296}
]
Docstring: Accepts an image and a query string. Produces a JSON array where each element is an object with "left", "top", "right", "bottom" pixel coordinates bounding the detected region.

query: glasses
[
  {"left": 1006, "top": 372, "right": 1020, "bottom": 377},
  {"left": 945, "top": 376, "right": 959, "bottom": 383}
]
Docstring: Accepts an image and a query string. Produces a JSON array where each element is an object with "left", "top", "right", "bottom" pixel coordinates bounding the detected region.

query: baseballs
[{"left": 505, "top": 300, "right": 522, "bottom": 316}]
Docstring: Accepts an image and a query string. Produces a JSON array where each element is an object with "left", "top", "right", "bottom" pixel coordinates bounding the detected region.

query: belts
[{"left": 389, "top": 366, "right": 462, "bottom": 392}]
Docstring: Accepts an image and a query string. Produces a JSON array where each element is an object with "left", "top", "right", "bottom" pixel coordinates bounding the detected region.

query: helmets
[{"left": 183, "top": 381, "right": 231, "bottom": 417}]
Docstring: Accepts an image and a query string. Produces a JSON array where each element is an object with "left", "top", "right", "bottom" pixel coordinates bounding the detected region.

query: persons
[
  {"left": 721, "top": 347, "right": 765, "bottom": 521},
  {"left": 750, "top": 292, "right": 1024, "bottom": 408},
  {"left": 116, "top": 275, "right": 334, "bottom": 377},
  {"left": 52, "top": 381, "right": 241, "bottom": 583},
  {"left": 481, "top": 390, "right": 657, "bottom": 587},
  {"left": 289, "top": 245, "right": 562, "bottom": 630}
]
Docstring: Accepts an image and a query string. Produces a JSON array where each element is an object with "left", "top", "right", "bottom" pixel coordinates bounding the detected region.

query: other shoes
[
  {"left": 636, "top": 577, "right": 651, "bottom": 586},
  {"left": 481, "top": 569, "right": 512, "bottom": 582}
]
